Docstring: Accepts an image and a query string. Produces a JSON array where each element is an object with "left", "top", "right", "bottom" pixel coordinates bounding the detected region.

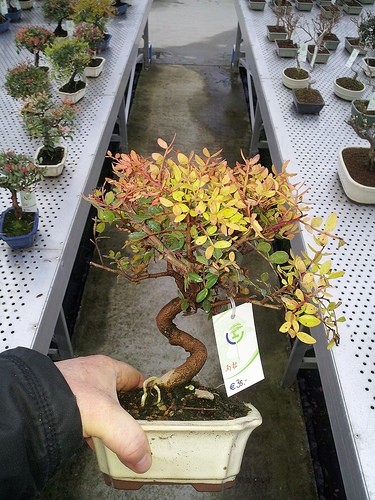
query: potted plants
[
  {"left": 275, "top": 11, "right": 305, "bottom": 58},
  {"left": 291, "top": 78, "right": 326, "bottom": 117},
  {"left": 265, "top": 2, "right": 293, "bottom": 43},
  {"left": 336, "top": 98, "right": 375, "bottom": 207},
  {"left": 76, "top": 129, "right": 349, "bottom": 492},
  {"left": 2, "top": 56, "right": 53, "bottom": 122},
  {"left": 0, "top": 147, "right": 51, "bottom": 251},
  {"left": 17, "top": 90, "right": 80, "bottom": 179},
  {"left": 43, "top": 34, "right": 94, "bottom": 107},
  {"left": 333, "top": 61, "right": 367, "bottom": 103},
  {"left": 282, "top": 52, "right": 310, "bottom": 89},
  {"left": 310, "top": 4, "right": 342, "bottom": 51},
  {"left": 296, "top": 16, "right": 340, "bottom": 64},
  {"left": 71, "top": 23, "right": 106, "bottom": 79},
  {"left": 13, "top": 23, "right": 55, "bottom": 75},
  {"left": 351, "top": 65, "right": 375, "bottom": 126},
  {"left": 38, "top": 0, "right": 76, "bottom": 39},
  {"left": 357, "top": 16, "right": 375, "bottom": 80},
  {"left": 67, "top": 0, "right": 116, "bottom": 52},
  {"left": 344, "top": 9, "right": 375, "bottom": 56},
  {"left": 4, "top": 0, "right": 23, "bottom": 24},
  {"left": 110, "top": 0, "right": 128, "bottom": 17}
]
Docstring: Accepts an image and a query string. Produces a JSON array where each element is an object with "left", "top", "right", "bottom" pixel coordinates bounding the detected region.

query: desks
[{"left": 0, "top": 0, "right": 375, "bottom": 500}]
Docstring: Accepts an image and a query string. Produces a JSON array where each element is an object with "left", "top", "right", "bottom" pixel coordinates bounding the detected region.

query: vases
[
  {"left": 272, "top": 0, "right": 293, "bottom": 15},
  {"left": 18, "top": 0, "right": 34, "bottom": 11},
  {"left": 342, "top": 0, "right": 364, "bottom": 16},
  {"left": 319, "top": 4, "right": 342, "bottom": 20},
  {"left": 295, "top": 0, "right": 314, "bottom": 12},
  {"left": 316, "top": 0, "right": 333, "bottom": 8},
  {"left": 0, "top": 14, "right": 11, "bottom": 34},
  {"left": 358, "top": 0, "right": 374, "bottom": 5},
  {"left": 249, "top": 0, "right": 267, "bottom": 12}
]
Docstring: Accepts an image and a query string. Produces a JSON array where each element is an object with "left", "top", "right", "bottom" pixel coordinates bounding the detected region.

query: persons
[{"left": 0, "top": 346, "right": 153, "bottom": 500}]
